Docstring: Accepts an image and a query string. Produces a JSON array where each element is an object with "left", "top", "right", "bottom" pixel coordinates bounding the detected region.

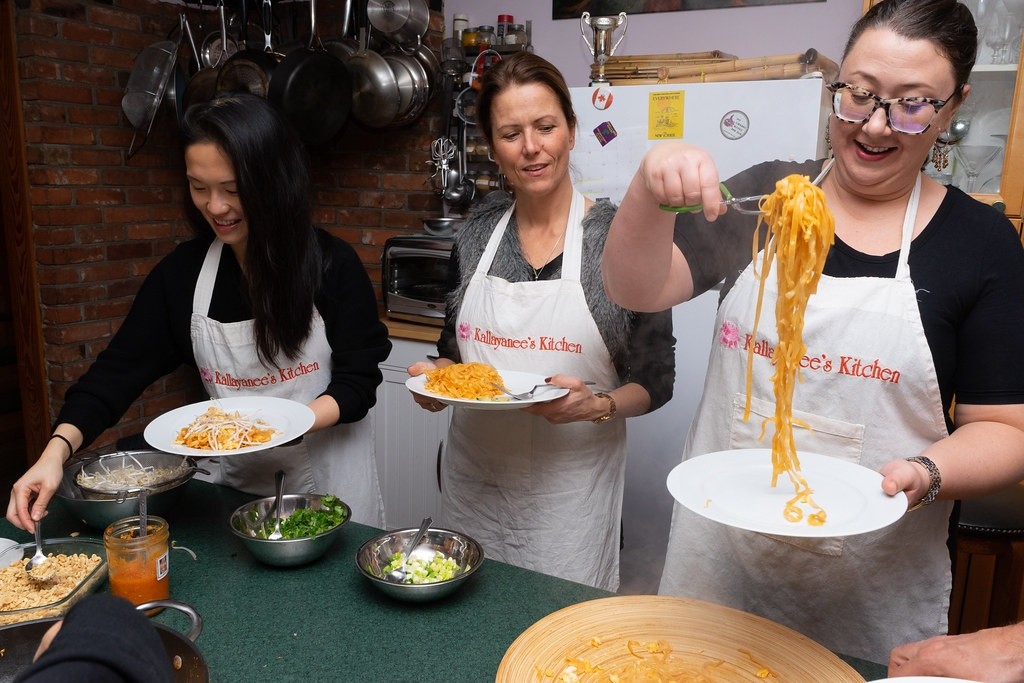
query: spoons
[
  {"left": 270, "top": 470, "right": 288, "bottom": 540},
  {"left": 382, "top": 518, "right": 434, "bottom": 584},
  {"left": 25, "top": 498, "right": 56, "bottom": 582}
]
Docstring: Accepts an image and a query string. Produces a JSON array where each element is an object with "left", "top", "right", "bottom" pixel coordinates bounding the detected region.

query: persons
[
  {"left": 406, "top": 50, "right": 677, "bottom": 592},
  {"left": 5, "top": 92, "right": 393, "bottom": 534},
  {"left": 601, "top": 1, "right": 1024, "bottom": 683},
  {"left": 10, "top": 593, "right": 171, "bottom": 683}
]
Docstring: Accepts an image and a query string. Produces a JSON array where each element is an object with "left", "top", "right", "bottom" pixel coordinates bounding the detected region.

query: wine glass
[{"left": 922, "top": 1, "right": 1024, "bottom": 194}]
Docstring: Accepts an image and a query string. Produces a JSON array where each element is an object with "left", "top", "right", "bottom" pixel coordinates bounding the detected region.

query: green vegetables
[{"left": 259, "top": 492, "right": 348, "bottom": 541}]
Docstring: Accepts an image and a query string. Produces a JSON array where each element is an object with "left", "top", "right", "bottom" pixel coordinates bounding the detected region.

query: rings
[{"left": 431, "top": 403, "right": 436, "bottom": 410}]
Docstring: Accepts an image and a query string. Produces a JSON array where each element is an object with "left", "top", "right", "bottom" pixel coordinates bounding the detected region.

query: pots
[
  {"left": 122, "top": 1, "right": 439, "bottom": 164},
  {"left": 0, "top": 600, "right": 211, "bottom": 683}
]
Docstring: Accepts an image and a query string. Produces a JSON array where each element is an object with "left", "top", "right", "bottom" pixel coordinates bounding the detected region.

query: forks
[{"left": 488, "top": 381, "right": 598, "bottom": 400}]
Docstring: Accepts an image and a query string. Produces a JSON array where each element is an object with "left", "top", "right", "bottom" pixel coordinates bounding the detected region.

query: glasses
[{"left": 825, "top": 81, "right": 964, "bottom": 135}]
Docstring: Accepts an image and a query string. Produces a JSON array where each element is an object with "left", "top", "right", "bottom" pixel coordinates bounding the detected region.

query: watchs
[{"left": 595, "top": 392, "right": 616, "bottom": 425}]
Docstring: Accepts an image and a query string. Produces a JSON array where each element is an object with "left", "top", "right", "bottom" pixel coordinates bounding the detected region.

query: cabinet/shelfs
[
  {"left": 371, "top": 368, "right": 453, "bottom": 532},
  {"left": 444, "top": 41, "right": 528, "bottom": 201},
  {"left": 860, "top": 1, "right": 1024, "bottom": 637}
]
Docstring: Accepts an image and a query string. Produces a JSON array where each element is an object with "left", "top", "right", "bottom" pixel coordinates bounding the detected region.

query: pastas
[
  {"left": 741, "top": 174, "right": 828, "bottom": 526},
  {"left": 533, "top": 638, "right": 782, "bottom": 683},
  {"left": 423, "top": 361, "right": 506, "bottom": 402},
  {"left": 177, "top": 408, "right": 274, "bottom": 451}
]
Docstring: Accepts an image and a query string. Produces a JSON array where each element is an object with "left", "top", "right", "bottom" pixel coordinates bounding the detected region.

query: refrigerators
[{"left": 563, "top": 77, "right": 836, "bottom": 600}]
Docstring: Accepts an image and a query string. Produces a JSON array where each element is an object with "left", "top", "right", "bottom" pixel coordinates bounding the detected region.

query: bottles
[{"left": 452, "top": 12, "right": 527, "bottom": 190}]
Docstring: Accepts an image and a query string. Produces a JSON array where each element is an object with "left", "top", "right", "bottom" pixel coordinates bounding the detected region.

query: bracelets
[
  {"left": 51, "top": 434, "right": 73, "bottom": 462},
  {"left": 905, "top": 456, "right": 942, "bottom": 512}
]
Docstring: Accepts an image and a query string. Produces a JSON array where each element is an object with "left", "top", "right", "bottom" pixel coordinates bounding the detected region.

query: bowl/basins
[
  {"left": 1, "top": 537, "right": 108, "bottom": 626},
  {"left": 355, "top": 528, "right": 484, "bottom": 601},
  {"left": 228, "top": 493, "right": 352, "bottom": 566},
  {"left": 420, "top": 217, "right": 464, "bottom": 236},
  {"left": 58, "top": 450, "right": 197, "bottom": 530}
]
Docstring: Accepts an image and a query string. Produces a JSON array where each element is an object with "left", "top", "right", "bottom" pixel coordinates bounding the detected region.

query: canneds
[{"left": 101, "top": 515, "right": 169, "bottom": 616}]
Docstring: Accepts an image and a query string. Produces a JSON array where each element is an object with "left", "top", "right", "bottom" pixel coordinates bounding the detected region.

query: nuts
[{"left": 1, "top": 531, "right": 103, "bottom": 628}]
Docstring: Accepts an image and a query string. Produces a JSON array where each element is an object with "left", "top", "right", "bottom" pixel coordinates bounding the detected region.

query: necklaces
[{"left": 515, "top": 210, "right": 566, "bottom": 280}]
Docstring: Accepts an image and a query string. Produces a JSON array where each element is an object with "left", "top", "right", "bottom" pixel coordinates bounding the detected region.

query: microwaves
[{"left": 381, "top": 236, "right": 457, "bottom": 326}]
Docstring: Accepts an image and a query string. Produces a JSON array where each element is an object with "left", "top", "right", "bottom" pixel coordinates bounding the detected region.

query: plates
[
  {"left": 667, "top": 448, "right": 909, "bottom": 538},
  {"left": 406, "top": 371, "right": 570, "bottom": 409},
  {"left": 143, "top": 397, "right": 316, "bottom": 456},
  {"left": 1, "top": 538, "right": 24, "bottom": 570}
]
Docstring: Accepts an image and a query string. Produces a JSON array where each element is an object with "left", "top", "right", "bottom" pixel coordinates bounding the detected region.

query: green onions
[{"left": 382, "top": 551, "right": 469, "bottom": 584}]
